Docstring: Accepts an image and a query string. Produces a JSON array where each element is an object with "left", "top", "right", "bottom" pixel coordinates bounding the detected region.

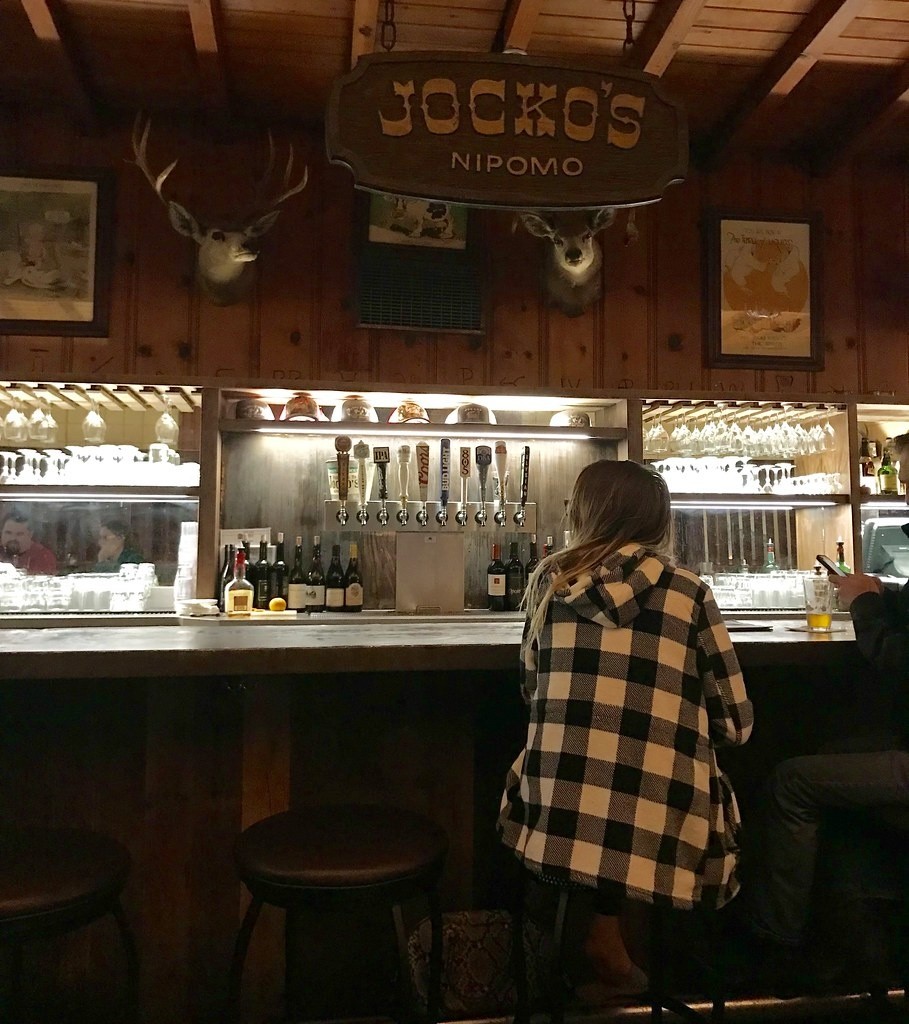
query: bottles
[
  {"left": 219, "top": 533, "right": 364, "bottom": 617},
  {"left": 487, "top": 531, "right": 574, "bottom": 611},
  {"left": 763, "top": 543, "right": 780, "bottom": 573},
  {"left": 834, "top": 542, "right": 851, "bottom": 574},
  {"left": 859, "top": 438, "right": 874, "bottom": 477},
  {"left": 878, "top": 447, "right": 898, "bottom": 495}
]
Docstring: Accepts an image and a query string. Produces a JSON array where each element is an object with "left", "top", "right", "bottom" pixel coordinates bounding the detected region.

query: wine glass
[
  {"left": 82, "top": 393, "right": 106, "bottom": 443},
  {"left": 155, "top": 394, "right": 179, "bottom": 445},
  {"left": 644, "top": 403, "right": 844, "bottom": 495},
  {"left": 0, "top": 391, "right": 59, "bottom": 443},
  {"left": 0, "top": 444, "right": 200, "bottom": 486}
]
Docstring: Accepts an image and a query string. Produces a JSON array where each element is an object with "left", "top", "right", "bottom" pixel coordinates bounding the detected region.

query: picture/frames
[
  {"left": 705, "top": 205, "right": 825, "bottom": 373},
  {"left": 0, "top": 160, "right": 115, "bottom": 338}
]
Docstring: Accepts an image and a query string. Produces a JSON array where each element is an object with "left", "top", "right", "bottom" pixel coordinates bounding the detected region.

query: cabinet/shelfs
[
  {"left": 595, "top": 399, "right": 909, "bottom": 620},
  {"left": 0, "top": 387, "right": 229, "bottom": 627}
]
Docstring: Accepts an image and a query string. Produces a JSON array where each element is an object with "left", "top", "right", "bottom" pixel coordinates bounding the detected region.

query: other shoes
[
  {"left": 575, "top": 965, "right": 652, "bottom": 1003},
  {"left": 692, "top": 950, "right": 796, "bottom": 996}
]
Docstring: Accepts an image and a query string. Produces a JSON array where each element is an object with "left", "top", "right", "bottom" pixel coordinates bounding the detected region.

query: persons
[
  {"left": 495, "top": 458, "right": 753, "bottom": 1002},
  {"left": 690, "top": 433, "right": 909, "bottom": 986},
  {"left": 0, "top": 511, "right": 57, "bottom": 575},
  {"left": 87, "top": 519, "right": 143, "bottom": 573}
]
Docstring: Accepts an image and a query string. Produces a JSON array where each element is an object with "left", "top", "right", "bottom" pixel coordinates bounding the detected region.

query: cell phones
[{"left": 816, "top": 554, "right": 848, "bottom": 577}]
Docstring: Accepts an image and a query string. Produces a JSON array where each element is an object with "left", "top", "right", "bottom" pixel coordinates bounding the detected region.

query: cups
[
  {"left": 0, "top": 521, "right": 199, "bottom": 612},
  {"left": 698, "top": 569, "right": 839, "bottom": 609},
  {"left": 803, "top": 579, "right": 834, "bottom": 631}
]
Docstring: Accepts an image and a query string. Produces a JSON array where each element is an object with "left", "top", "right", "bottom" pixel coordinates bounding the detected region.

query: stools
[
  {"left": 1, "top": 827, "right": 141, "bottom": 1024},
  {"left": 222, "top": 800, "right": 450, "bottom": 1024}
]
